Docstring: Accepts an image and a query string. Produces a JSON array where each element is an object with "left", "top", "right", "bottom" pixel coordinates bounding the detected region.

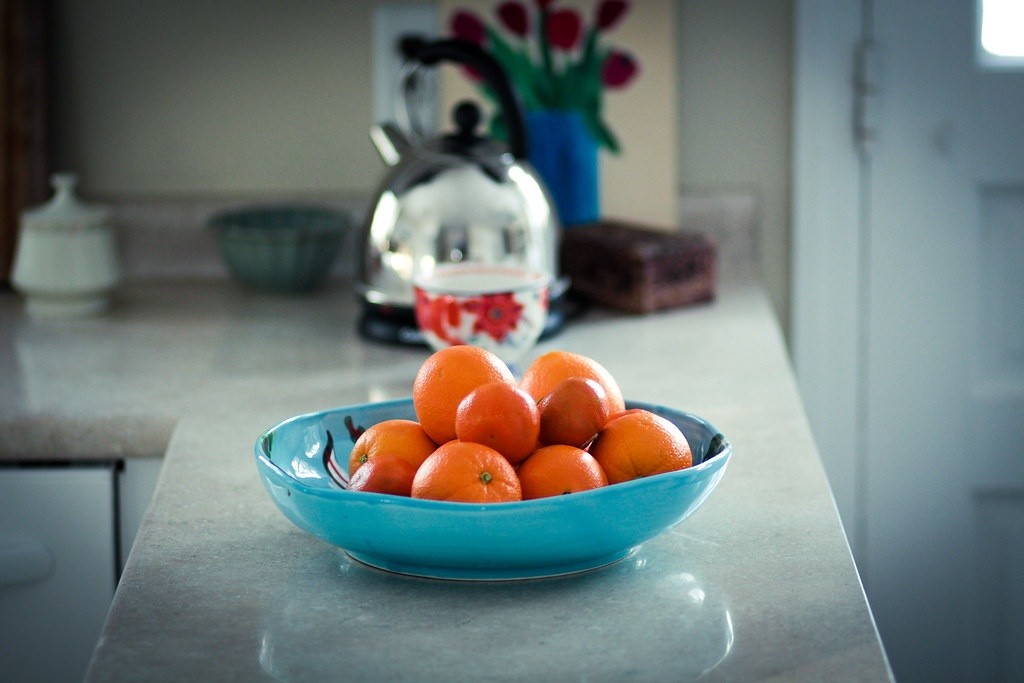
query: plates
[{"left": 254, "top": 397, "right": 732, "bottom": 580}]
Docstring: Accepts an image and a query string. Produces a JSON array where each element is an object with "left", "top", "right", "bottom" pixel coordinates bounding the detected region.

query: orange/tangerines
[{"left": 347, "top": 345, "right": 693, "bottom": 503}]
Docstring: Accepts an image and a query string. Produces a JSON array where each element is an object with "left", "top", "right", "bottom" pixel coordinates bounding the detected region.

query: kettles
[{"left": 359, "top": 39, "right": 570, "bottom": 351}]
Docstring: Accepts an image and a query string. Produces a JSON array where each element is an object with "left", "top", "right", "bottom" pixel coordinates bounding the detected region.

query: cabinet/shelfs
[{"left": 0, "top": 455, "right": 168, "bottom": 683}]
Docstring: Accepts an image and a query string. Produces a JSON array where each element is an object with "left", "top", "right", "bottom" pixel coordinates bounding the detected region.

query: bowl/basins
[{"left": 211, "top": 208, "right": 348, "bottom": 294}]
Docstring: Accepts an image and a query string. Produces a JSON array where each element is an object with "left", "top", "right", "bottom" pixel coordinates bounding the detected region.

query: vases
[{"left": 501, "top": 102, "right": 601, "bottom": 231}]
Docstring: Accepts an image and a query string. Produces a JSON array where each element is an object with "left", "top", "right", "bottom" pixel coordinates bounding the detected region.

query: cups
[
  {"left": 13, "top": 172, "right": 117, "bottom": 320},
  {"left": 415, "top": 265, "right": 547, "bottom": 362}
]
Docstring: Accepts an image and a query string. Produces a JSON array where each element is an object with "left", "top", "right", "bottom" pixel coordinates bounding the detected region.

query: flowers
[{"left": 448, "top": 0, "right": 641, "bottom": 157}]
